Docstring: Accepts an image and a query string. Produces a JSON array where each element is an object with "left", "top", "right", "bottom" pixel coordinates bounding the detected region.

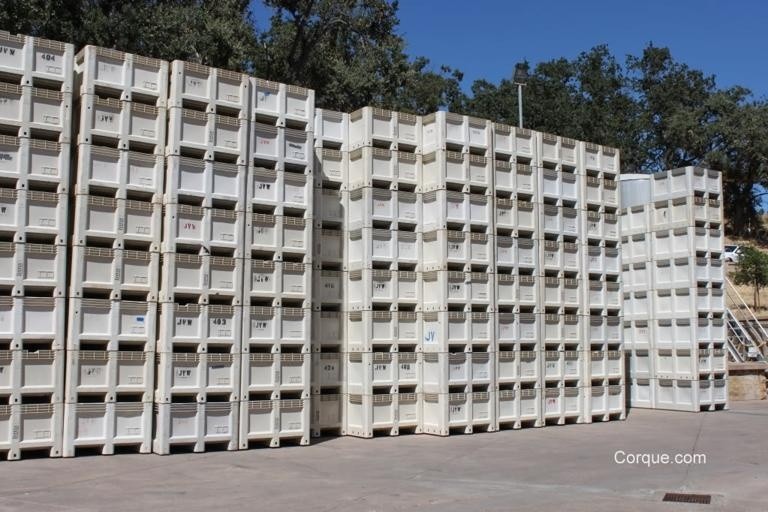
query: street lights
[{"left": 512, "top": 62, "right": 530, "bottom": 129}]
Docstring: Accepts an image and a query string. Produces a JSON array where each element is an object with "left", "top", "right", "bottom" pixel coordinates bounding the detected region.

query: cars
[{"left": 724, "top": 244, "right": 745, "bottom": 263}]
